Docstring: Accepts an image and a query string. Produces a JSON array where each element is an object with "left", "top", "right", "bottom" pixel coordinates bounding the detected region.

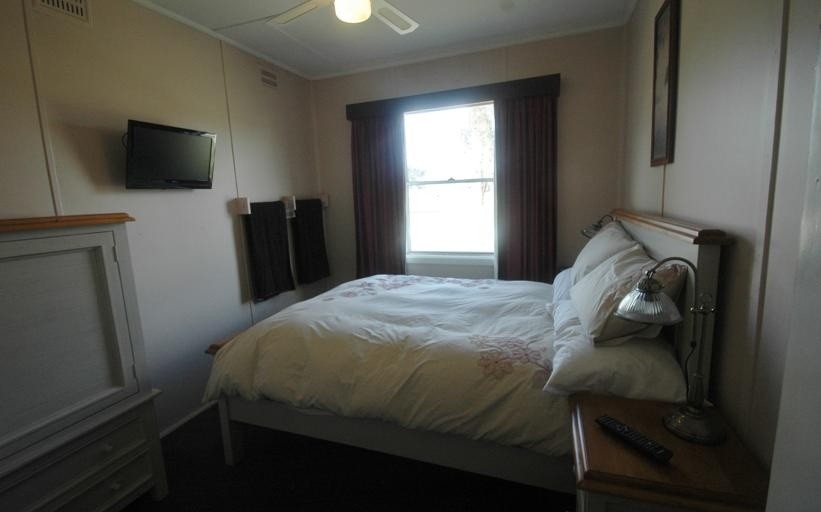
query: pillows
[
  {"left": 573, "top": 219, "right": 641, "bottom": 284},
  {"left": 551, "top": 264, "right": 575, "bottom": 305},
  {"left": 575, "top": 243, "right": 689, "bottom": 346},
  {"left": 543, "top": 298, "right": 718, "bottom": 411}
]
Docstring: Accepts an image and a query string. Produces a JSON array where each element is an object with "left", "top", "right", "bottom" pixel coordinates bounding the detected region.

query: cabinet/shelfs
[{"left": 1, "top": 210, "right": 173, "bottom": 510}]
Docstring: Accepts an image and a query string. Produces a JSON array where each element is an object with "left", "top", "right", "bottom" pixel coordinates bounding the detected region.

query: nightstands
[{"left": 568, "top": 393, "right": 769, "bottom": 510}]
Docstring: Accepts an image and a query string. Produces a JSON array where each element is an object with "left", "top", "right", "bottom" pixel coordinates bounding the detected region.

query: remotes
[{"left": 595, "top": 413, "right": 673, "bottom": 463}]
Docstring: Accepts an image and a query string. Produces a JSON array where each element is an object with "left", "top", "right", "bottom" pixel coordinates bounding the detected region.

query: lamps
[
  {"left": 617, "top": 254, "right": 729, "bottom": 446},
  {"left": 581, "top": 213, "right": 614, "bottom": 240}
]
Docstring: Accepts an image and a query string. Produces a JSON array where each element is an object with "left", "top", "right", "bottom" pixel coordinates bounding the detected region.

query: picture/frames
[{"left": 649, "top": 1, "right": 683, "bottom": 166}]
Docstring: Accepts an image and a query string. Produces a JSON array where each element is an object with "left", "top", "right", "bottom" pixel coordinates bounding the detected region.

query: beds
[{"left": 201, "top": 208, "right": 737, "bottom": 494}]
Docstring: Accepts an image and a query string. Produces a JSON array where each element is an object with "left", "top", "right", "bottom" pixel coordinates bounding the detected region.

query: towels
[
  {"left": 240, "top": 199, "right": 299, "bottom": 305},
  {"left": 289, "top": 196, "right": 335, "bottom": 288}
]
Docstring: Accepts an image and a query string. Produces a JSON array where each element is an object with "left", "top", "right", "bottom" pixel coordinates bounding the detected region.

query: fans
[{"left": 268, "top": 0, "right": 420, "bottom": 37}]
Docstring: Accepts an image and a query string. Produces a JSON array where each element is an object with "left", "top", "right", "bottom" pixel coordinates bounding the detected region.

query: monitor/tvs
[{"left": 126, "top": 119, "right": 216, "bottom": 189}]
